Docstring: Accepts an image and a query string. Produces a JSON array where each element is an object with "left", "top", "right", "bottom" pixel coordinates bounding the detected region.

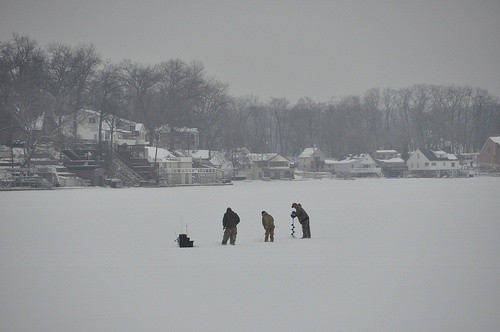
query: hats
[
  {"left": 262, "top": 211, "right": 267, "bottom": 215},
  {"left": 292, "top": 203, "right": 297, "bottom": 208},
  {"left": 227, "top": 208, "right": 231, "bottom": 212}
]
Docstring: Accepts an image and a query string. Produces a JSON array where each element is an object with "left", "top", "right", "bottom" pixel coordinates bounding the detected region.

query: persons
[
  {"left": 291, "top": 202, "right": 311, "bottom": 238},
  {"left": 261, "top": 211, "right": 274, "bottom": 242},
  {"left": 220, "top": 206, "right": 240, "bottom": 245}
]
